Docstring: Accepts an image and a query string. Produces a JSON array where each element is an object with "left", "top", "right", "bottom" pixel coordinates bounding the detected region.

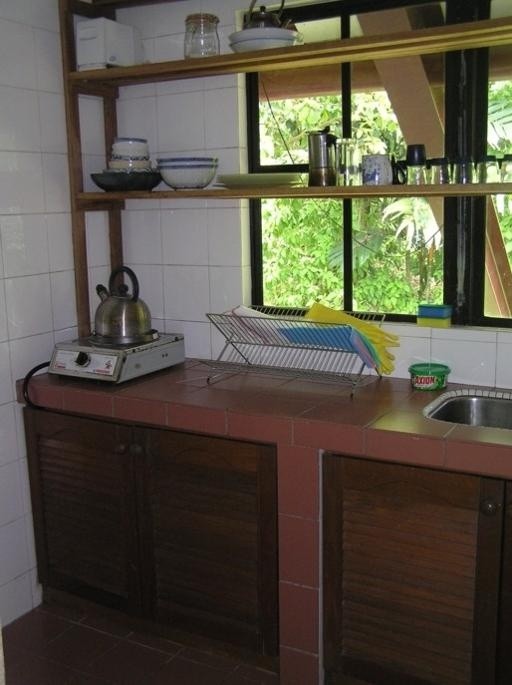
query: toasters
[{"left": 74, "top": 18, "right": 143, "bottom": 70}]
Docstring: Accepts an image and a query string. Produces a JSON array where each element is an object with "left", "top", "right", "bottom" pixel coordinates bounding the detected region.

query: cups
[
  {"left": 334, "top": 137, "right": 361, "bottom": 187},
  {"left": 183, "top": 12, "right": 219, "bottom": 60}
]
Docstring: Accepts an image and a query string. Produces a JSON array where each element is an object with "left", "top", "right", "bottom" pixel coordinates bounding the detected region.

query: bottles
[{"left": 358, "top": 143, "right": 512, "bottom": 186}]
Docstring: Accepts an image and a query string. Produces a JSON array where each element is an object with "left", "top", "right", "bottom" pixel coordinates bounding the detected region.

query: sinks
[{"left": 422, "top": 388, "right": 512, "bottom": 430}]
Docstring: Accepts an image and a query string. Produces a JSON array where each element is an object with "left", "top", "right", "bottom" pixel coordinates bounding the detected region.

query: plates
[{"left": 215, "top": 170, "right": 305, "bottom": 188}]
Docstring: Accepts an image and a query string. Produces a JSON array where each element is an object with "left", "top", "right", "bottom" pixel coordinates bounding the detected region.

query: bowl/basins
[
  {"left": 227, "top": 28, "right": 300, "bottom": 55},
  {"left": 158, "top": 156, "right": 219, "bottom": 192},
  {"left": 89, "top": 172, "right": 162, "bottom": 195},
  {"left": 102, "top": 136, "right": 157, "bottom": 172}
]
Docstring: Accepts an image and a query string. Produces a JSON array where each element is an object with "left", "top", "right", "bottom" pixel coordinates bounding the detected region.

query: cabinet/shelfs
[
  {"left": 320, "top": 453, "right": 512, "bottom": 685},
  {"left": 58, "top": 0, "right": 512, "bottom": 340},
  {"left": 21, "top": 406, "right": 279, "bottom": 657}
]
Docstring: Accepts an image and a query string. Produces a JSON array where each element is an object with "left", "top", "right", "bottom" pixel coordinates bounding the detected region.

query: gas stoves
[{"left": 47, "top": 328, "right": 187, "bottom": 383}]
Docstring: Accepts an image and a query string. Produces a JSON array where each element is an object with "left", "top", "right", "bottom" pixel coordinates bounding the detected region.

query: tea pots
[
  {"left": 241, "top": 0, "right": 293, "bottom": 30},
  {"left": 93, "top": 265, "right": 152, "bottom": 338},
  {"left": 306, "top": 126, "right": 338, "bottom": 187}
]
